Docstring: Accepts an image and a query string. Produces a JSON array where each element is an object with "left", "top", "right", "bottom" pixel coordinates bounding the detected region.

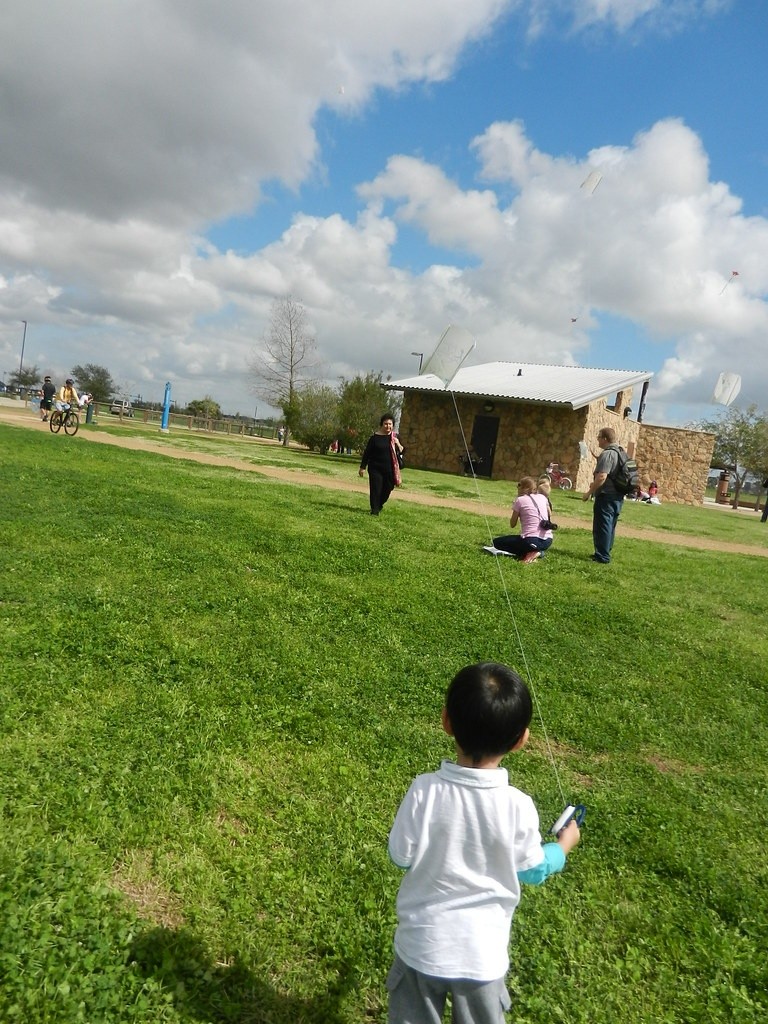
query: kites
[
  {"left": 719, "top": 270, "right": 740, "bottom": 294},
  {"left": 712, "top": 370, "right": 743, "bottom": 406},
  {"left": 417, "top": 321, "right": 475, "bottom": 387},
  {"left": 579, "top": 171, "right": 610, "bottom": 194}
]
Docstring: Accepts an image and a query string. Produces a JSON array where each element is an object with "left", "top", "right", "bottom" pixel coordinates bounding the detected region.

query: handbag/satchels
[{"left": 397, "top": 451, "right": 405, "bottom": 469}]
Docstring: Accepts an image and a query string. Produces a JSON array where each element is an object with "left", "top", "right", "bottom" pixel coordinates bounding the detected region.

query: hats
[
  {"left": 45, "top": 376, "right": 51, "bottom": 381},
  {"left": 66, "top": 379, "right": 74, "bottom": 384}
]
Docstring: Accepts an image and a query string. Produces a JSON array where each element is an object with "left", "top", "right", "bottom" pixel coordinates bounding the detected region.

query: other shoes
[
  {"left": 67, "top": 420, "right": 74, "bottom": 426},
  {"left": 590, "top": 554, "right": 597, "bottom": 560},
  {"left": 522, "top": 551, "right": 541, "bottom": 564},
  {"left": 42, "top": 416, "right": 48, "bottom": 422}
]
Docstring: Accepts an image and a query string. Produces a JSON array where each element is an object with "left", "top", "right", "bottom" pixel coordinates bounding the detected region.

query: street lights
[
  {"left": 17, "top": 320, "right": 28, "bottom": 385},
  {"left": 411, "top": 351, "right": 424, "bottom": 376}
]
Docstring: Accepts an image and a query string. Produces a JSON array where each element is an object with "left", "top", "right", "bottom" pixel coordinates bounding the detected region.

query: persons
[
  {"left": 331, "top": 440, "right": 352, "bottom": 454},
  {"left": 278, "top": 425, "right": 284, "bottom": 442},
  {"left": 40, "top": 376, "right": 56, "bottom": 421},
  {"left": 628, "top": 480, "right": 658, "bottom": 503},
  {"left": 582, "top": 427, "right": 624, "bottom": 563},
  {"left": 460, "top": 445, "right": 480, "bottom": 477},
  {"left": 53, "top": 378, "right": 80, "bottom": 426},
  {"left": 387, "top": 661, "right": 581, "bottom": 1024},
  {"left": 76, "top": 392, "right": 93, "bottom": 424},
  {"left": 357, "top": 413, "right": 405, "bottom": 515},
  {"left": 493, "top": 475, "right": 553, "bottom": 565}
]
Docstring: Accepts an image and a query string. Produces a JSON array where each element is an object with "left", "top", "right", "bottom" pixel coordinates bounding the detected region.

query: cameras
[{"left": 540, "top": 520, "right": 557, "bottom": 531}]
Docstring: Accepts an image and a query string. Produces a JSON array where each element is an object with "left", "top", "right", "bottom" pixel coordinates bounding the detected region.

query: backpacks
[{"left": 606, "top": 446, "right": 638, "bottom": 494}]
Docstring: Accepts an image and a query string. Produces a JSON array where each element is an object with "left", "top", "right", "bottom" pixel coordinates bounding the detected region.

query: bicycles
[{"left": 50, "top": 402, "right": 82, "bottom": 436}]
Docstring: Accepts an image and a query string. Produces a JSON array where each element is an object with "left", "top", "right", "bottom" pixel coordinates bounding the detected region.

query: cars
[
  {"left": 0, "top": 381, "right": 7, "bottom": 392},
  {"left": 110, "top": 397, "right": 135, "bottom": 417}
]
